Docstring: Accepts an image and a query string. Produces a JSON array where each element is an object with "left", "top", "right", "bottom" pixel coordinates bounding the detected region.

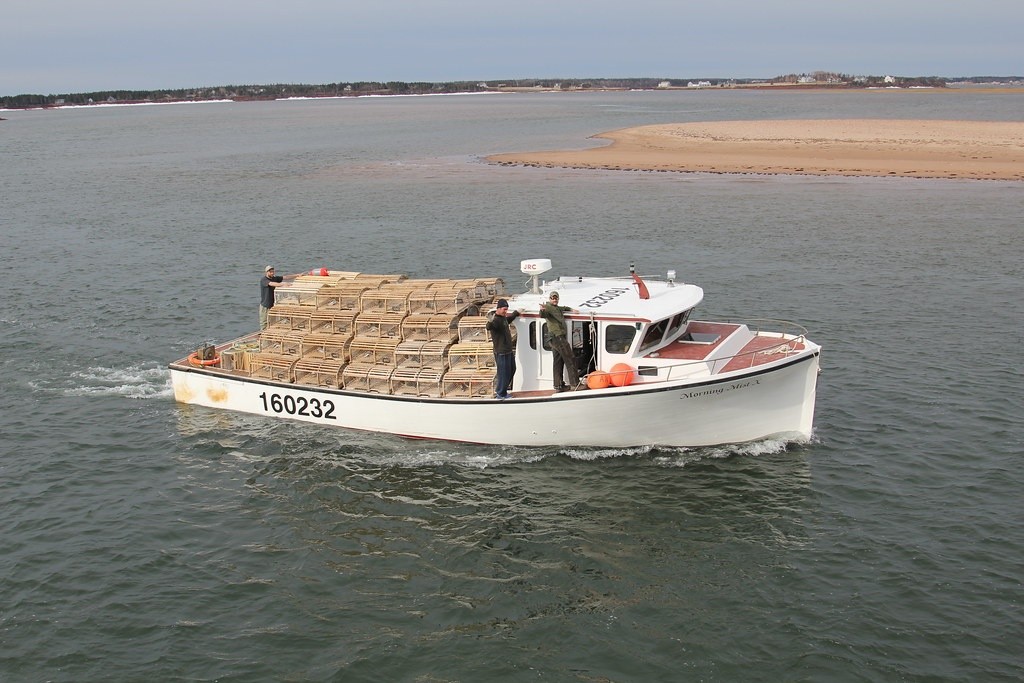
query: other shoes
[
  {"left": 496, "top": 393, "right": 513, "bottom": 398},
  {"left": 575, "top": 384, "right": 588, "bottom": 390},
  {"left": 556, "top": 385, "right": 570, "bottom": 393}
]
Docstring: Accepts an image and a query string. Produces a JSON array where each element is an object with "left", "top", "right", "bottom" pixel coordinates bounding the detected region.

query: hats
[
  {"left": 497, "top": 300, "right": 509, "bottom": 308},
  {"left": 265, "top": 266, "right": 274, "bottom": 272},
  {"left": 550, "top": 292, "right": 559, "bottom": 298}
]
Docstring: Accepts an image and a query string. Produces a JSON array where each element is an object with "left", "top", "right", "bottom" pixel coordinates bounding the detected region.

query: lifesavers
[{"left": 187, "top": 352, "right": 220, "bottom": 365}]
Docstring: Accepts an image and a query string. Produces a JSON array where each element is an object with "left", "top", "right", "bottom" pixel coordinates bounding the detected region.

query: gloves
[
  {"left": 486, "top": 312, "right": 495, "bottom": 322},
  {"left": 518, "top": 308, "right": 525, "bottom": 313}
]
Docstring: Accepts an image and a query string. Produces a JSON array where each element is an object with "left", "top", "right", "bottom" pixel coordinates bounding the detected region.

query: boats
[{"left": 168, "top": 259, "right": 823, "bottom": 452}]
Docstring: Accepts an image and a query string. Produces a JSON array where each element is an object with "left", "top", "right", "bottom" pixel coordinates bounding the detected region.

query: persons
[
  {"left": 539, "top": 291, "right": 588, "bottom": 393},
  {"left": 258, "top": 266, "right": 308, "bottom": 332},
  {"left": 486, "top": 299, "right": 525, "bottom": 399}
]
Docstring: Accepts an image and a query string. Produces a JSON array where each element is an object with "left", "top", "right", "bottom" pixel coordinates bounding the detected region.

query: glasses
[{"left": 550, "top": 297, "right": 558, "bottom": 300}]
